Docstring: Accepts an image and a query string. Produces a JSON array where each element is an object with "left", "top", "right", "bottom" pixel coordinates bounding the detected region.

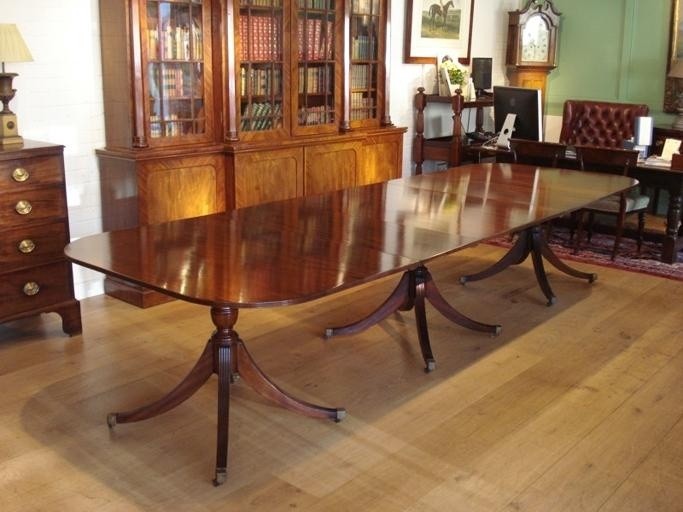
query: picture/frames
[{"left": 403, "top": 1, "right": 474, "bottom": 65}]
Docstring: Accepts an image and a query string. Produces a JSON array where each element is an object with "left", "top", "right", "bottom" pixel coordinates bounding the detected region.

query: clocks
[{"left": 508, "top": 1, "right": 562, "bottom": 68}]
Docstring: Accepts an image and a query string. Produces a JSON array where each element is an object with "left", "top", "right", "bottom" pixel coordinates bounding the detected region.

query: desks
[
  {"left": 477, "top": 131, "right": 683, "bottom": 265},
  {"left": 63, "top": 161, "right": 640, "bottom": 488}
]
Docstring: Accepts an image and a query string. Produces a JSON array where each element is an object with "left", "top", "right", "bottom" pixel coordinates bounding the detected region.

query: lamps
[{"left": 0, "top": 24, "right": 33, "bottom": 147}]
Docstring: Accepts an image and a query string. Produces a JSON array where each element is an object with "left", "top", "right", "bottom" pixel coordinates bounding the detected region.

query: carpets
[{"left": 484, "top": 216, "right": 683, "bottom": 281}]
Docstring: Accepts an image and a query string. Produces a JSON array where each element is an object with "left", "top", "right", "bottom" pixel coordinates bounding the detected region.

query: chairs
[
  {"left": 559, "top": 99, "right": 649, "bottom": 148},
  {"left": 507, "top": 138, "right": 567, "bottom": 246},
  {"left": 569, "top": 145, "right": 650, "bottom": 262}
]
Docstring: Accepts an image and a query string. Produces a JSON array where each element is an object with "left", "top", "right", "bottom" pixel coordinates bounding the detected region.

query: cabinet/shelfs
[
  {"left": 510, "top": 70, "right": 550, "bottom": 136},
  {"left": 98, "top": 0, "right": 408, "bottom": 230},
  {"left": 0, "top": 140, "right": 83, "bottom": 340},
  {"left": 412, "top": 87, "right": 494, "bottom": 175}
]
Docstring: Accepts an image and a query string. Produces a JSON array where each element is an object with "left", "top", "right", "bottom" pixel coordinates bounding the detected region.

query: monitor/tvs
[{"left": 493, "top": 86, "right": 543, "bottom": 149}]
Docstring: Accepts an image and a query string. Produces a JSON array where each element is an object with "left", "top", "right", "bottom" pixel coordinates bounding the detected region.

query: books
[{"left": 147, "top": 0, "right": 378, "bottom": 137}]
